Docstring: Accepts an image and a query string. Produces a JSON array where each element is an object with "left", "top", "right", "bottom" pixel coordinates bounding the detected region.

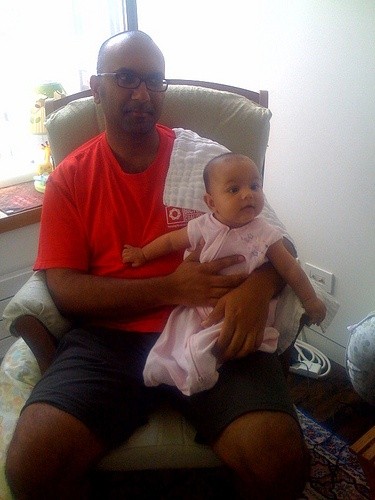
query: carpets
[{"left": 292, "top": 399, "right": 375, "bottom": 500}]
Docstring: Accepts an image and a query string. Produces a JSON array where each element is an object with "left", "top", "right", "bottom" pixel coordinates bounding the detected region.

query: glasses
[{"left": 97, "top": 72, "right": 170, "bottom": 93}]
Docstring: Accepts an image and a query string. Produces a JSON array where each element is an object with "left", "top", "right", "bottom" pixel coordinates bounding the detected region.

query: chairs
[{"left": 2, "top": 79, "right": 339, "bottom": 500}]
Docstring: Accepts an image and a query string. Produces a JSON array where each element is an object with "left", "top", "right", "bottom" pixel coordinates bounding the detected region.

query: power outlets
[{"left": 304, "top": 263, "right": 334, "bottom": 294}]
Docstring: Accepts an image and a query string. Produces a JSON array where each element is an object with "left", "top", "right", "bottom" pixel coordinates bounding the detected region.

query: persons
[
  {"left": 121, "top": 153, "right": 328, "bottom": 396},
  {"left": 4, "top": 30, "right": 311, "bottom": 500}
]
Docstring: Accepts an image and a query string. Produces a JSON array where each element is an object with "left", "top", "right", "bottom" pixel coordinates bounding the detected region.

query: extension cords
[{"left": 289, "top": 360, "right": 322, "bottom": 380}]
